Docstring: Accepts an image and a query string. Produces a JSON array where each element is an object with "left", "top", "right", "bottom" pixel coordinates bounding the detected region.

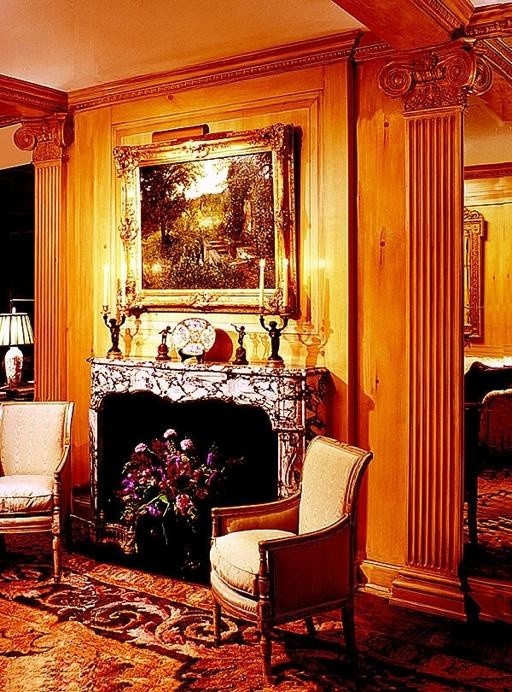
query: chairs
[
  {"left": 0, "top": 400, "right": 78, "bottom": 584},
  {"left": 207, "top": 434, "right": 376, "bottom": 683}
]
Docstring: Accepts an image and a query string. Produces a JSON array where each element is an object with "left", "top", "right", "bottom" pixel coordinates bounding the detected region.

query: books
[{"left": 0, "top": 382, "right": 35, "bottom": 401}]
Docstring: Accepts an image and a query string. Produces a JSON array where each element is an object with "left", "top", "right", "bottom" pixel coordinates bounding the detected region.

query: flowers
[{"left": 113, "top": 423, "right": 248, "bottom": 549}]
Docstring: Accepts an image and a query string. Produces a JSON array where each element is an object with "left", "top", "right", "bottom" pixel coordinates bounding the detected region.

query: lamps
[{"left": 0, "top": 306, "right": 35, "bottom": 387}]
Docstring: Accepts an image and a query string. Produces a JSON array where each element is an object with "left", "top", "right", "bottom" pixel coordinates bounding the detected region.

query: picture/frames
[{"left": 112, "top": 120, "right": 303, "bottom": 318}]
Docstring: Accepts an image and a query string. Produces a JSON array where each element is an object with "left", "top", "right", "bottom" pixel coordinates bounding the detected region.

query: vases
[{"left": 136, "top": 502, "right": 193, "bottom": 571}]
